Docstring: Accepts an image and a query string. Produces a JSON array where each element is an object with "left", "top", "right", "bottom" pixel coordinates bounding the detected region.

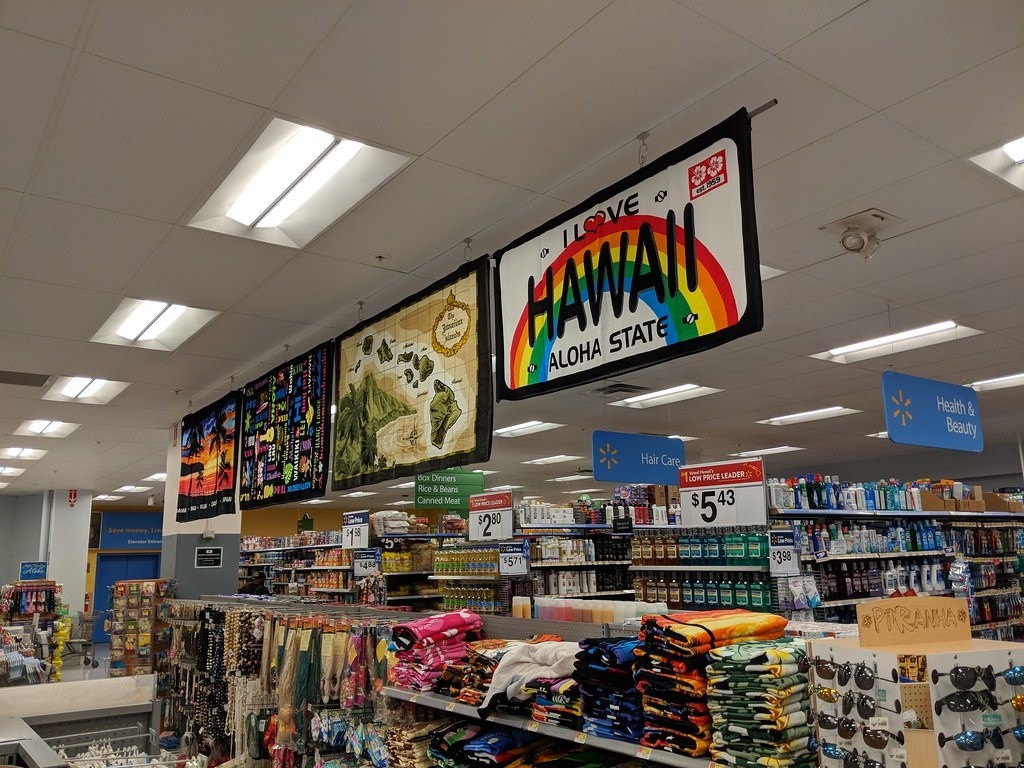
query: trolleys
[{"left": 49, "top": 607, "right": 105, "bottom": 668}]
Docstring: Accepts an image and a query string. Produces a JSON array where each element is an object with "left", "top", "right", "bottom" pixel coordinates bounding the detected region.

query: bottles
[
  {"left": 767, "top": 473, "right": 933, "bottom": 511},
  {"left": 416, "top": 518, "right": 430, "bottom": 534},
  {"left": 631, "top": 529, "right": 770, "bottom": 566},
  {"left": 802, "top": 519, "right": 947, "bottom": 555},
  {"left": 601, "top": 502, "right": 681, "bottom": 526},
  {"left": 443, "top": 583, "right": 500, "bottom": 611},
  {"left": 532, "top": 536, "right": 631, "bottom": 563},
  {"left": 434, "top": 545, "right": 499, "bottom": 576},
  {"left": 632, "top": 571, "right": 772, "bottom": 614},
  {"left": 241, "top": 536, "right": 287, "bottom": 550},
  {"left": 534, "top": 569, "right": 632, "bottom": 594},
  {"left": 806, "top": 559, "right": 946, "bottom": 601}
]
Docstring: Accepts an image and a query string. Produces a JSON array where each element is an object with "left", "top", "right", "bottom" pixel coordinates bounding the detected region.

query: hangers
[{"left": 52, "top": 737, "right": 140, "bottom": 768}]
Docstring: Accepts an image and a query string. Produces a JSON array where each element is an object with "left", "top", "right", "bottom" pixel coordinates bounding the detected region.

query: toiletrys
[
  {"left": 520, "top": 500, "right": 575, "bottom": 525},
  {"left": 597, "top": 569, "right": 635, "bottom": 592},
  {"left": 530, "top": 539, "right": 595, "bottom": 563},
  {"left": 633, "top": 571, "right": 773, "bottom": 613},
  {"left": 512, "top": 596, "right": 668, "bottom": 623},
  {"left": 594, "top": 534, "right": 632, "bottom": 561},
  {"left": 631, "top": 525, "right": 770, "bottom": 566},
  {"left": 601, "top": 503, "right": 668, "bottom": 525}
]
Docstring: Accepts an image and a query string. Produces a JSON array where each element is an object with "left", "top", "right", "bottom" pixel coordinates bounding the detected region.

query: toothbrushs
[
  {"left": 945, "top": 529, "right": 1024, "bottom": 555},
  {"left": 971, "top": 564, "right": 996, "bottom": 588},
  {"left": 981, "top": 592, "right": 1022, "bottom": 620},
  {"left": 973, "top": 627, "right": 1014, "bottom": 641}
]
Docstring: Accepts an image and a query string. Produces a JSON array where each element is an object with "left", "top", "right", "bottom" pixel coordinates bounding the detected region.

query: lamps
[{"left": 837, "top": 225, "right": 882, "bottom": 263}]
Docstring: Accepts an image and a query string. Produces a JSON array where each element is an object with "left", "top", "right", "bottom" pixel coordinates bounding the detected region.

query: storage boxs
[
  {"left": 955, "top": 499, "right": 986, "bottom": 512},
  {"left": 921, "top": 489, "right": 955, "bottom": 511},
  {"left": 648, "top": 485, "right": 665, "bottom": 506},
  {"left": 664, "top": 486, "right": 679, "bottom": 508},
  {"left": 982, "top": 492, "right": 1022, "bottom": 512}
]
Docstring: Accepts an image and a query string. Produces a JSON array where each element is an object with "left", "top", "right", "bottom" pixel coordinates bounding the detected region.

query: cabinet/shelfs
[
  {"left": 513, "top": 523, "right": 635, "bottom": 599},
  {"left": 770, "top": 508, "right": 1024, "bottom": 610},
  {"left": 373, "top": 533, "right": 468, "bottom": 600},
  {"left": 307, "top": 544, "right": 357, "bottom": 593},
  {"left": 427, "top": 540, "right": 510, "bottom": 616},
  {"left": 238, "top": 549, "right": 275, "bottom": 580},
  {"left": 271, "top": 546, "right": 313, "bottom": 586}
]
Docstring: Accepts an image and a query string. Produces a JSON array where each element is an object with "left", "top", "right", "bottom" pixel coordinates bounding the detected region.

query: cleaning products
[
  {"left": 826, "top": 604, "right": 858, "bottom": 624},
  {"left": 768, "top": 473, "right": 922, "bottom": 511},
  {"left": 533, "top": 570, "right": 596, "bottom": 595},
  {"left": 773, "top": 518, "right": 947, "bottom": 556},
  {"left": 806, "top": 558, "right": 945, "bottom": 601}
]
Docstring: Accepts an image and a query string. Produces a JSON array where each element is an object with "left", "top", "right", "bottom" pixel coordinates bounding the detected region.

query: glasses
[
  {"left": 797, "top": 654, "right": 904, "bottom": 768},
  {"left": 931, "top": 666, "right": 1024, "bottom": 768}
]
